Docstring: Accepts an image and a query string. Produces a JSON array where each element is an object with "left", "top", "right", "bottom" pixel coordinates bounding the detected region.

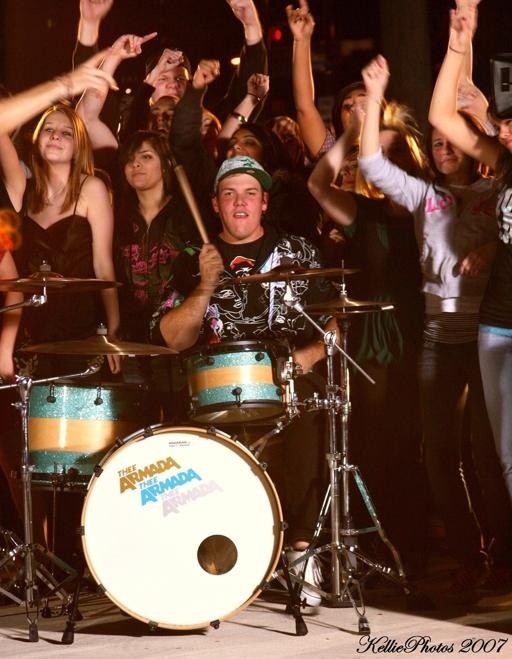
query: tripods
[
  {"left": 0, "top": 390, "right": 95, "bottom": 641},
  {"left": 269, "top": 344, "right": 418, "bottom": 633},
  {"left": 0, "top": 526, "right": 85, "bottom": 622},
  {"left": 295, "top": 328, "right": 414, "bottom": 596}
]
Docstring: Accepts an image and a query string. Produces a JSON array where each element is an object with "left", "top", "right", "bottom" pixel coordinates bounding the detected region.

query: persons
[{"left": 1, "top": 0, "right": 512, "bottom": 616}]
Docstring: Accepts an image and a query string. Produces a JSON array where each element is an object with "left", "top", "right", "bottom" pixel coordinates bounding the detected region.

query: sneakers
[{"left": 284, "top": 548, "right": 323, "bottom": 608}]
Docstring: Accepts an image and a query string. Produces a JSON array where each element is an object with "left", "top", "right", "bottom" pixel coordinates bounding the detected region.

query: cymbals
[
  {"left": 303, "top": 297, "right": 398, "bottom": 318},
  {"left": 239, "top": 265, "right": 361, "bottom": 284},
  {"left": 17, "top": 334, "right": 179, "bottom": 358},
  {"left": 0, "top": 271, "right": 124, "bottom": 299}
]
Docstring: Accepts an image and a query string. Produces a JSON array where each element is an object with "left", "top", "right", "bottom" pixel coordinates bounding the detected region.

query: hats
[{"left": 214, "top": 154, "right": 272, "bottom": 195}]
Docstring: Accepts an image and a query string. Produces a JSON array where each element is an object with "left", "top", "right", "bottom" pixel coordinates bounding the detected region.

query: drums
[
  {"left": 184, "top": 340, "right": 286, "bottom": 423},
  {"left": 79, "top": 421, "right": 286, "bottom": 635},
  {"left": 22, "top": 382, "right": 158, "bottom": 490}
]
